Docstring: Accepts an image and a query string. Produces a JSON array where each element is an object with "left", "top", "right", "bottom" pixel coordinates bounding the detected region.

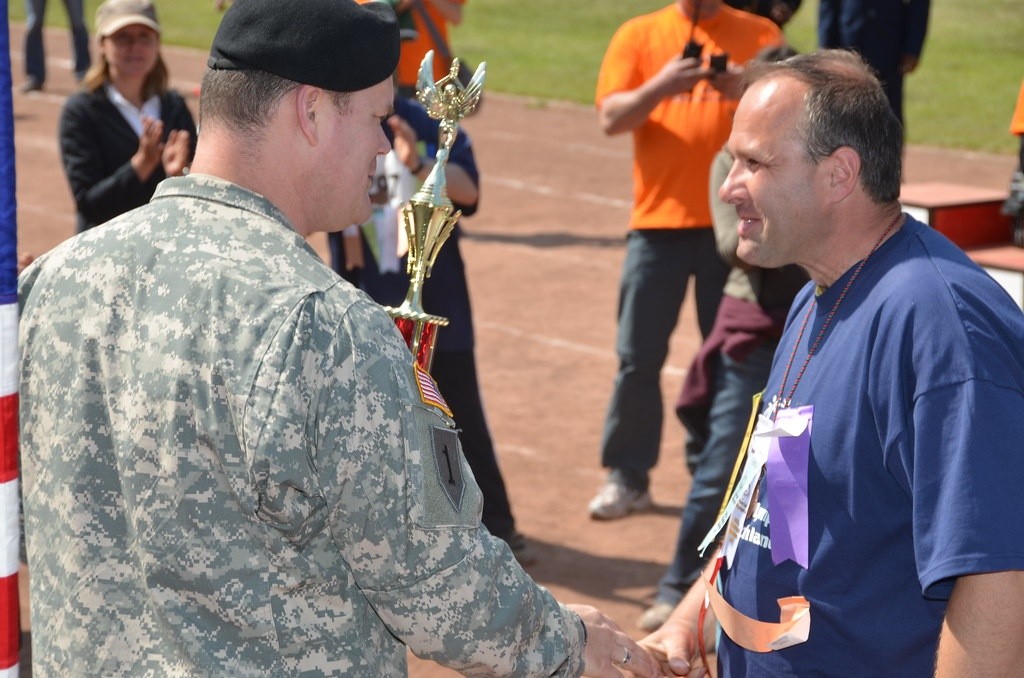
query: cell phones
[{"left": 710, "top": 54, "right": 728, "bottom": 73}]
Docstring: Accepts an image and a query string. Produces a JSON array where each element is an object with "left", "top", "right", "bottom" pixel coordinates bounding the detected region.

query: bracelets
[{"left": 410, "top": 155, "right": 425, "bottom": 175}]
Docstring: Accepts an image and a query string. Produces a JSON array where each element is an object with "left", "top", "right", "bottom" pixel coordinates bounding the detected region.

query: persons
[
  {"left": 15, "top": 1, "right": 666, "bottom": 677},
  {"left": 16, "top": 0, "right": 935, "bottom": 633},
  {"left": 633, "top": 49, "right": 1023, "bottom": 678}
]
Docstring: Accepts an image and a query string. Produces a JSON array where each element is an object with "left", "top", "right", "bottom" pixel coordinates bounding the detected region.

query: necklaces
[{"left": 773, "top": 213, "right": 901, "bottom": 420}]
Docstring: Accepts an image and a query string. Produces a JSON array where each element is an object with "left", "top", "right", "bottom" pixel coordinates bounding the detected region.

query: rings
[{"left": 623, "top": 646, "right": 631, "bottom": 664}]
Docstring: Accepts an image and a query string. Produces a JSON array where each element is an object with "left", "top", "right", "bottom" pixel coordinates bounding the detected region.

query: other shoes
[
  {"left": 20, "top": 77, "right": 43, "bottom": 91},
  {"left": 73, "top": 71, "right": 87, "bottom": 82},
  {"left": 637, "top": 604, "right": 674, "bottom": 632},
  {"left": 503, "top": 531, "right": 536, "bottom": 565}
]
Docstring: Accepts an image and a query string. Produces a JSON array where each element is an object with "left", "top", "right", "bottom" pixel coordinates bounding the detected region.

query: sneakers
[{"left": 588, "top": 482, "right": 651, "bottom": 520}]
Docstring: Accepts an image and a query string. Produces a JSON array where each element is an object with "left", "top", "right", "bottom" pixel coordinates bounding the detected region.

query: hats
[
  {"left": 97, "top": 0, "right": 161, "bottom": 37},
  {"left": 208, "top": 0, "right": 402, "bottom": 93}
]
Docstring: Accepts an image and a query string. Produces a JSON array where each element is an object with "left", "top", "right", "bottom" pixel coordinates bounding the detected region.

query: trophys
[{"left": 381, "top": 48, "right": 488, "bottom": 374}]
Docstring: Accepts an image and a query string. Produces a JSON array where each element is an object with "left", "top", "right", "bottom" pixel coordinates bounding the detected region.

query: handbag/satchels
[{"left": 448, "top": 61, "right": 483, "bottom": 111}]
